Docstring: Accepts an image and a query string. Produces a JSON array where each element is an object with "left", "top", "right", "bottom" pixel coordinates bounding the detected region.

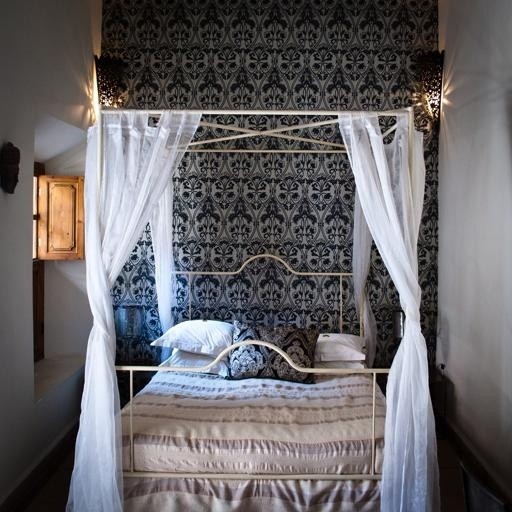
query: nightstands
[
  {"left": 375, "top": 370, "right": 448, "bottom": 440},
  {"left": 79, "top": 363, "right": 157, "bottom": 410}
]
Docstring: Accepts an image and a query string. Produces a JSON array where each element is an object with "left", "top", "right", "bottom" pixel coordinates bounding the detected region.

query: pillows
[{"left": 150, "top": 319, "right": 367, "bottom": 385}]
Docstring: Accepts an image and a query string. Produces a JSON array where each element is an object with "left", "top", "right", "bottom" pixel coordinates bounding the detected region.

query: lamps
[
  {"left": 94, "top": 53, "right": 128, "bottom": 108},
  {"left": 415, "top": 49, "right": 445, "bottom": 122}
]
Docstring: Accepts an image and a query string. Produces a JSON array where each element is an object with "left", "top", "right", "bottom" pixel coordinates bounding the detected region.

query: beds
[{"left": 115, "top": 254, "right": 391, "bottom": 512}]
